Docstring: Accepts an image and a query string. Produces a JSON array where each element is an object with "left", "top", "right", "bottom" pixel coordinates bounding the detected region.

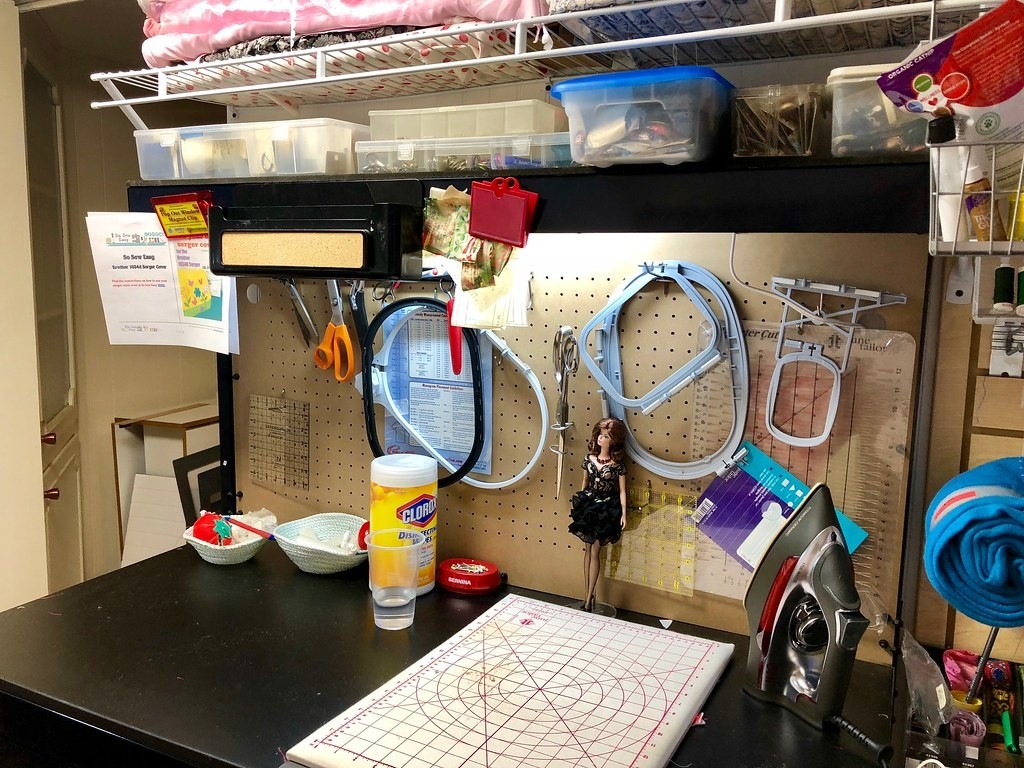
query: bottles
[
  {"left": 928, "top": 115, "right": 1007, "bottom": 243},
  {"left": 368, "top": 453, "right": 438, "bottom": 596}
]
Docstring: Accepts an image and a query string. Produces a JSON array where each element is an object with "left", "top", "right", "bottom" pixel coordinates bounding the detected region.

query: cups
[{"left": 363, "top": 528, "right": 426, "bottom": 631}]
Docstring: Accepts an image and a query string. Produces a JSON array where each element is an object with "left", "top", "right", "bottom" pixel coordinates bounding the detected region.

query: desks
[{"left": 0, "top": 535, "right": 901, "bottom": 768}]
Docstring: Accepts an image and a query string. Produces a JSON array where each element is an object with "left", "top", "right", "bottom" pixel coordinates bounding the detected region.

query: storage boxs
[
  {"left": 370, "top": 99, "right": 568, "bottom": 140},
  {"left": 825, "top": 63, "right": 926, "bottom": 156},
  {"left": 725, "top": 84, "right": 829, "bottom": 158},
  {"left": 550, "top": 65, "right": 737, "bottom": 167},
  {"left": 354, "top": 132, "right": 570, "bottom": 174},
  {"left": 133, "top": 117, "right": 367, "bottom": 182}
]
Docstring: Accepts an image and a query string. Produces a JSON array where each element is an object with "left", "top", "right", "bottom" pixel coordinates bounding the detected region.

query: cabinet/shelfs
[{"left": 90, "top": 0, "right": 1005, "bottom": 526}]
